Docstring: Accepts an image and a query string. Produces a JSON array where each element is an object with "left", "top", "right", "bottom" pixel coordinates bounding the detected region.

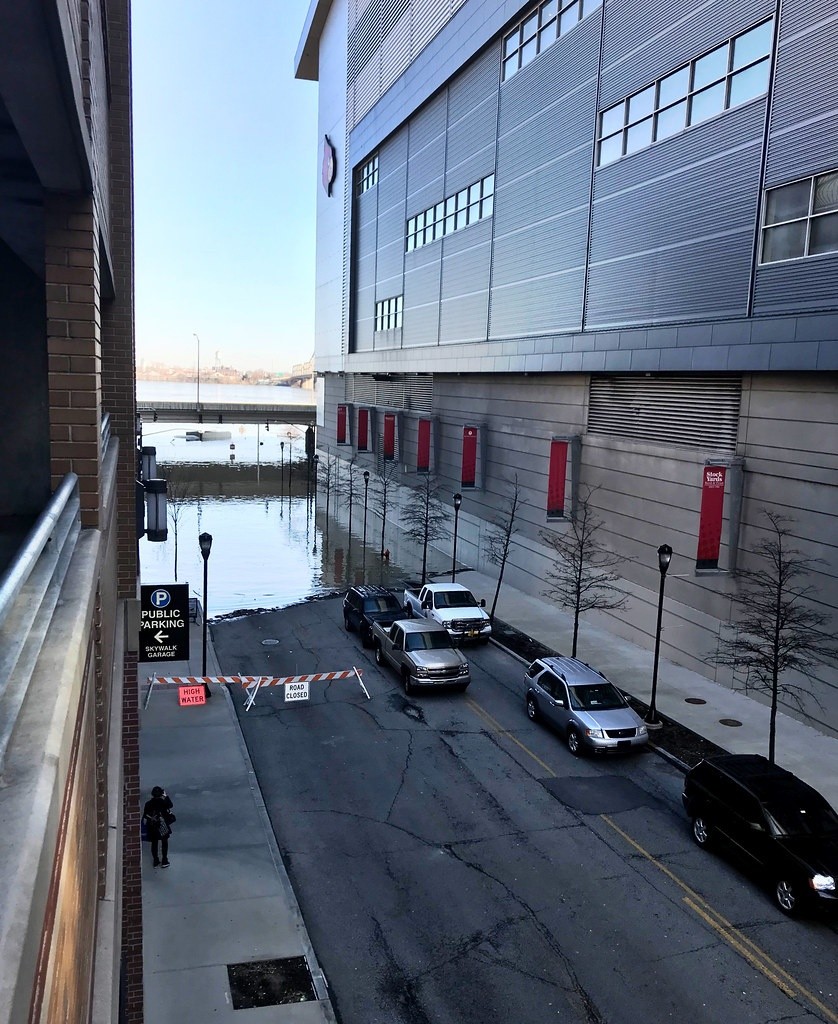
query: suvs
[
  {"left": 341, "top": 583, "right": 406, "bottom": 649},
  {"left": 523, "top": 655, "right": 650, "bottom": 755},
  {"left": 682, "top": 753, "right": 838, "bottom": 914}
]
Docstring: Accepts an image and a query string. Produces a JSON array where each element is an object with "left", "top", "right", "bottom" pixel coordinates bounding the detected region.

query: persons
[{"left": 144, "top": 786, "right": 174, "bottom": 868}]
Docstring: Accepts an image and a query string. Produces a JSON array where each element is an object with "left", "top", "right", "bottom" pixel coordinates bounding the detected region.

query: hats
[{"left": 151, "top": 786, "right": 163, "bottom": 796}]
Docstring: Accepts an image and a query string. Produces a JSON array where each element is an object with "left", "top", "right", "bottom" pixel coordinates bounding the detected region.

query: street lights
[
  {"left": 650, "top": 543, "right": 674, "bottom": 728},
  {"left": 451, "top": 492, "right": 463, "bottom": 582},
  {"left": 198, "top": 532, "right": 213, "bottom": 687}
]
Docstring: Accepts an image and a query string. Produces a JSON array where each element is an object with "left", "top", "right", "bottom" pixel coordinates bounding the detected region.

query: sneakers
[
  {"left": 153, "top": 857, "right": 161, "bottom": 869},
  {"left": 162, "top": 859, "right": 171, "bottom": 868}
]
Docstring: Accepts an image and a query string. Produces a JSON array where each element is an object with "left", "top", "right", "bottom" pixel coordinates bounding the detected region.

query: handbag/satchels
[
  {"left": 141, "top": 817, "right": 148, "bottom": 841},
  {"left": 164, "top": 813, "right": 176, "bottom": 824},
  {"left": 148, "top": 814, "right": 161, "bottom": 843},
  {"left": 158, "top": 817, "right": 172, "bottom": 842}
]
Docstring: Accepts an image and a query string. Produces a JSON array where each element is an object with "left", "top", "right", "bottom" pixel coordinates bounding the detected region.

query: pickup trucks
[
  {"left": 404, "top": 582, "right": 492, "bottom": 645},
  {"left": 371, "top": 618, "right": 472, "bottom": 695}
]
